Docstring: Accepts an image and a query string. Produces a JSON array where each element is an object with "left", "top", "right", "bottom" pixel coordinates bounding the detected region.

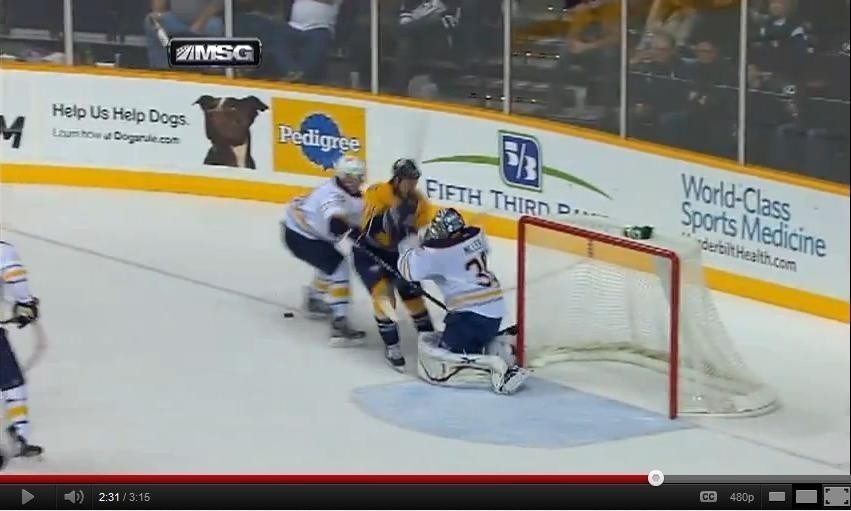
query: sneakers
[{"left": 497, "top": 364, "right": 530, "bottom": 396}]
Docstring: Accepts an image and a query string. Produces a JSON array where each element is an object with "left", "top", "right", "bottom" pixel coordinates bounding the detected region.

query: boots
[
  {"left": 6, "top": 426, "right": 42, "bottom": 459},
  {"left": 374, "top": 316, "right": 405, "bottom": 367},
  {"left": 304, "top": 286, "right": 335, "bottom": 314},
  {"left": 410, "top": 308, "right": 434, "bottom": 332},
  {"left": 329, "top": 315, "right": 367, "bottom": 340}
]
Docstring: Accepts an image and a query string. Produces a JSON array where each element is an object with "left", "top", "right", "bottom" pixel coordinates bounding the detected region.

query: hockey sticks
[{"left": 357, "top": 242, "right": 518, "bottom": 336}]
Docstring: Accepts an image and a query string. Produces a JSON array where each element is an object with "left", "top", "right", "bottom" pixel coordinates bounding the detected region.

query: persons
[
  {"left": 397, "top": 207, "right": 517, "bottom": 392},
  {"left": 0, "top": 241, "right": 44, "bottom": 471},
  {"left": 347, "top": 157, "right": 445, "bottom": 366},
  {"left": 276, "top": 153, "right": 367, "bottom": 339}
]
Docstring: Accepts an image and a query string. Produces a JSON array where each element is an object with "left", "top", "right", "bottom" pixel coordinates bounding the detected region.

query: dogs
[{"left": 193, "top": 95, "right": 271, "bottom": 172}]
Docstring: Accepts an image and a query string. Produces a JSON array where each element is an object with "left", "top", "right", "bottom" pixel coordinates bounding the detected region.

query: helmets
[
  {"left": 334, "top": 155, "right": 368, "bottom": 184},
  {"left": 392, "top": 157, "right": 420, "bottom": 179},
  {"left": 430, "top": 207, "right": 465, "bottom": 241}
]
